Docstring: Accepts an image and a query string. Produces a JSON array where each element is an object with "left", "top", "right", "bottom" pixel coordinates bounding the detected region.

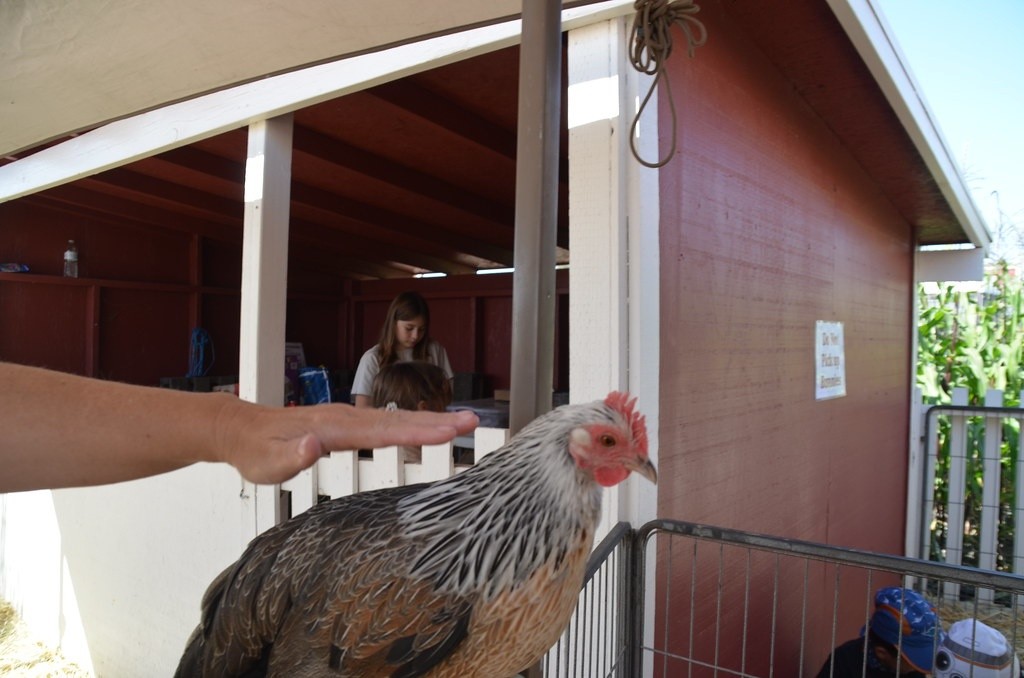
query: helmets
[{"left": 936, "top": 619, "right": 1020, "bottom": 678}]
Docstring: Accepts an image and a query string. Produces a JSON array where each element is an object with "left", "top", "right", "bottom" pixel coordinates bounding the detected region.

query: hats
[{"left": 860, "top": 587, "right": 945, "bottom": 674}]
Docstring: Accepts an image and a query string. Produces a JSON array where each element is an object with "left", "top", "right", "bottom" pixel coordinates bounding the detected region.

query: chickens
[{"left": 172, "top": 391, "right": 656, "bottom": 678}]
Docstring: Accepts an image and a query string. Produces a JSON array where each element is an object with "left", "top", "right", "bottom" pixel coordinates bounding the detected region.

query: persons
[
  {"left": 814, "top": 586, "right": 945, "bottom": 678},
  {"left": 0, "top": 362, "right": 480, "bottom": 494},
  {"left": 349, "top": 291, "right": 456, "bottom": 462},
  {"left": 933, "top": 618, "right": 1024, "bottom": 678}
]
